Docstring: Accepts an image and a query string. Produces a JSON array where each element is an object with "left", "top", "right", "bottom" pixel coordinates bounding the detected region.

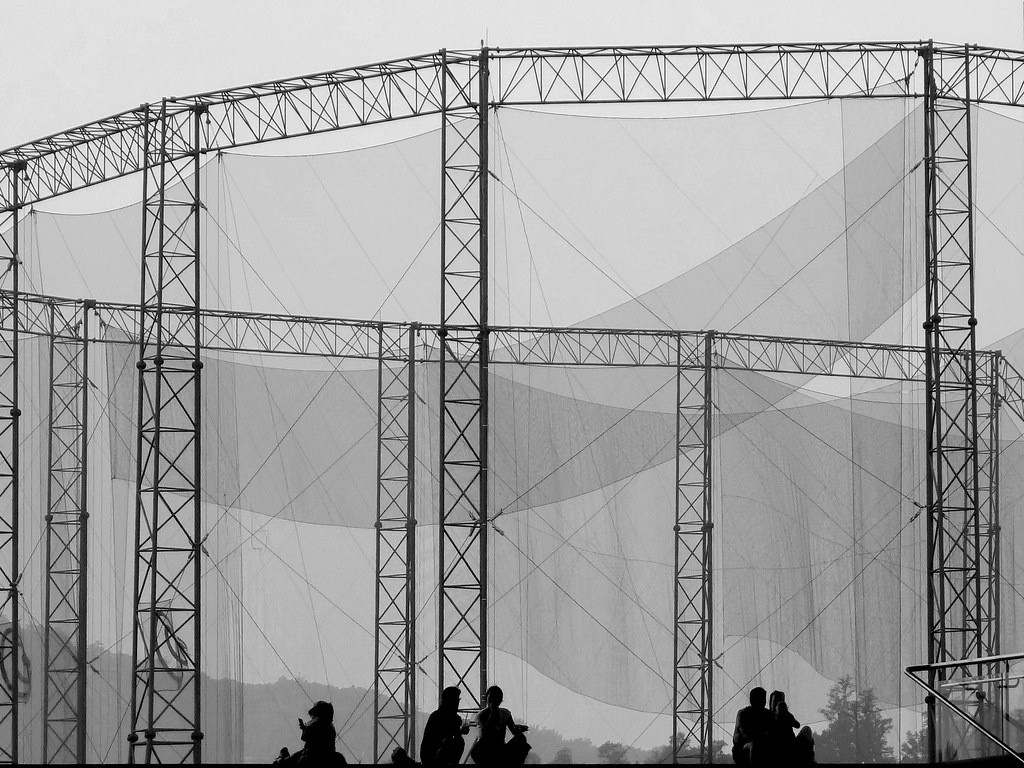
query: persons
[
  {"left": 296, "top": 701, "right": 337, "bottom": 764},
  {"left": 767, "top": 690, "right": 817, "bottom": 764},
  {"left": 471, "top": 686, "right": 530, "bottom": 765},
  {"left": 731, "top": 687, "right": 778, "bottom": 764},
  {"left": 420, "top": 686, "right": 469, "bottom": 766}
]
